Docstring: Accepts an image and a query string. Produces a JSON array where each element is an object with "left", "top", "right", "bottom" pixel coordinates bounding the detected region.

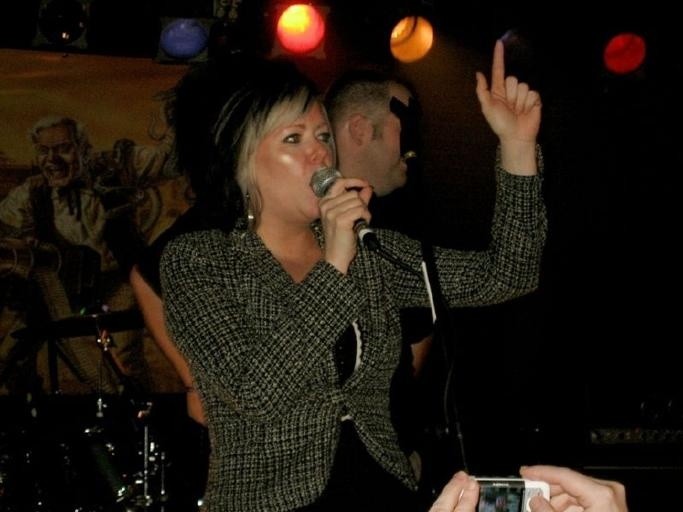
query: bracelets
[{"left": 181, "top": 381, "right": 198, "bottom": 391}]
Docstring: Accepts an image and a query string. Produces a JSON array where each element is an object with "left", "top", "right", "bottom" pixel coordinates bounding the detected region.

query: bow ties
[{"left": 57, "top": 177, "right": 87, "bottom": 223}]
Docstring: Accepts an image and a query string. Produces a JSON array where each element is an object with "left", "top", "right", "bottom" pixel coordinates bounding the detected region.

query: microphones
[{"left": 310, "top": 167, "right": 380, "bottom": 252}]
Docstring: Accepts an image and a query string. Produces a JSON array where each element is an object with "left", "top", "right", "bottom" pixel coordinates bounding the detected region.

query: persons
[
  {"left": 427, "top": 461, "right": 632, "bottom": 512},
  {"left": 0, "top": 115, "right": 176, "bottom": 396},
  {"left": 154, "top": 37, "right": 555, "bottom": 512},
  {"left": 128, "top": 63, "right": 436, "bottom": 512}
]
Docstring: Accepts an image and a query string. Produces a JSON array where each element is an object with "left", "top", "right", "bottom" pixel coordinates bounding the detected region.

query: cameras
[{"left": 457, "top": 477, "right": 551, "bottom": 512}]
